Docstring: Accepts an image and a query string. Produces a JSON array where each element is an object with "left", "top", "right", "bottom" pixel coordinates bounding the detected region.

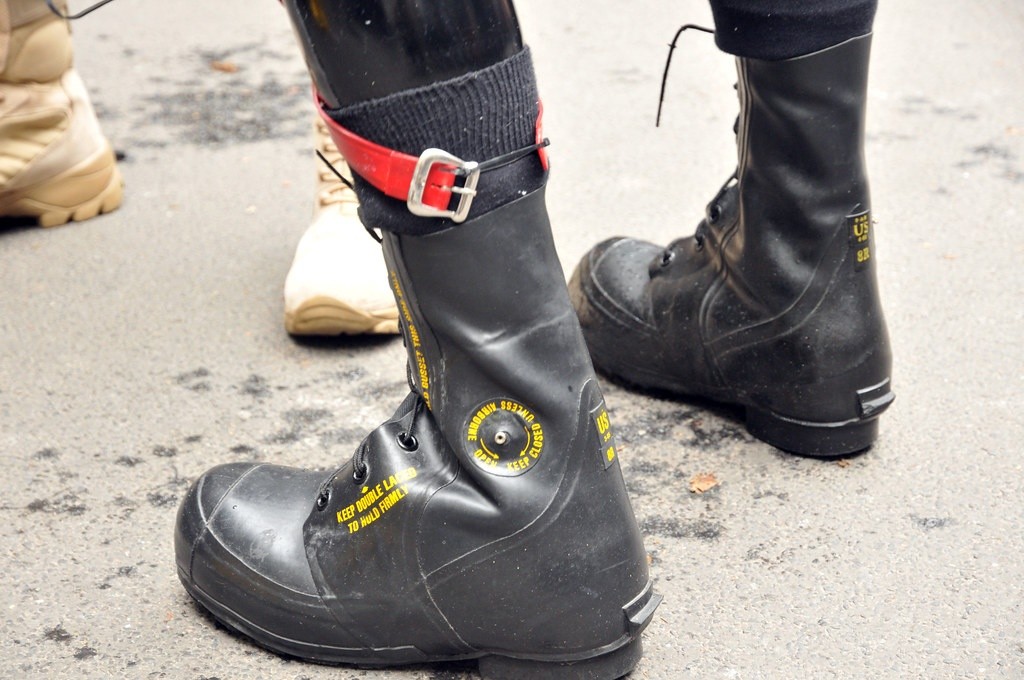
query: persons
[
  {"left": 171, "top": 0, "right": 900, "bottom": 679},
  {"left": 0, "top": 2, "right": 402, "bottom": 338}
]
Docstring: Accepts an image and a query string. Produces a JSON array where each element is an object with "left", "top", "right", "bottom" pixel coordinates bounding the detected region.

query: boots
[
  {"left": 176, "top": 186, "right": 662, "bottom": 680},
  {"left": 0, "top": 0, "right": 123, "bottom": 226},
  {"left": 568, "top": 32, "right": 894, "bottom": 457},
  {"left": 284, "top": 97, "right": 400, "bottom": 336}
]
